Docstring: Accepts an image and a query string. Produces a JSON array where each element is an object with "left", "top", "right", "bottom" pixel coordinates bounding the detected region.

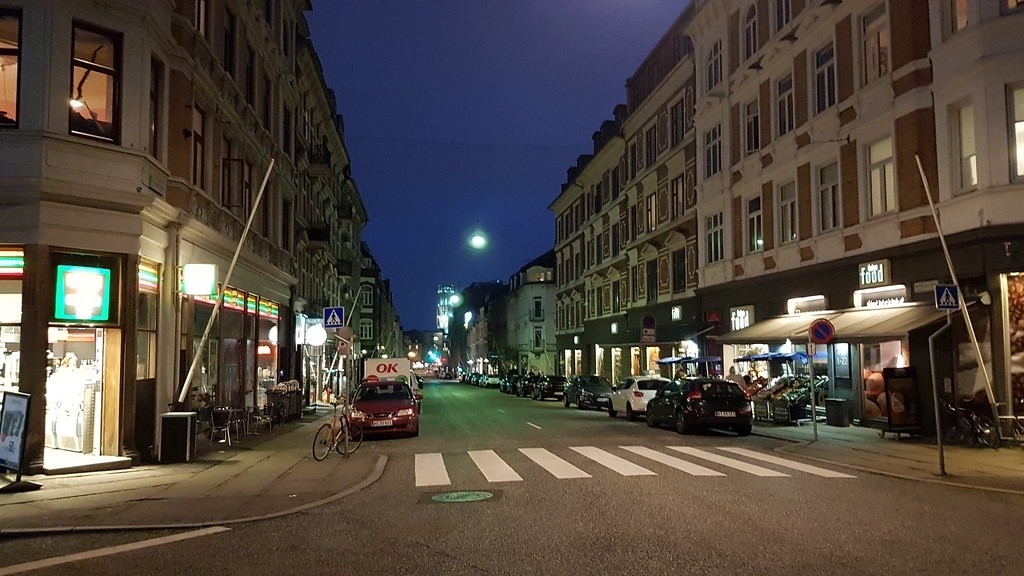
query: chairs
[
  {"left": 210, "top": 406, "right": 231, "bottom": 446},
  {"left": 226, "top": 409, "right": 247, "bottom": 440},
  {"left": 253, "top": 402, "right": 274, "bottom": 435}
]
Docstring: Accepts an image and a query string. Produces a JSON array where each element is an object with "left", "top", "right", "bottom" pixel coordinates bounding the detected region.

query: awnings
[{"left": 719, "top": 299, "right": 977, "bottom": 339}]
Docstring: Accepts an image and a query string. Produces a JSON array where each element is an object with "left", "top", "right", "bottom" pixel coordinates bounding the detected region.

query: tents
[{"left": 733, "top": 352, "right": 828, "bottom": 390}]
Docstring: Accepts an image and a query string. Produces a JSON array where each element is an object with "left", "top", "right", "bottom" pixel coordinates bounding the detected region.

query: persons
[
  {"left": 701, "top": 363, "right": 718, "bottom": 379},
  {"left": 674, "top": 367, "right": 687, "bottom": 381},
  {"left": 726, "top": 366, "right": 752, "bottom": 393}
]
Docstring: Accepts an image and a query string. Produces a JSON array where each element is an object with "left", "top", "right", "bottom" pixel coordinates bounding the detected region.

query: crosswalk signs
[
  {"left": 322, "top": 307, "right": 344, "bottom": 328},
  {"left": 934, "top": 284, "right": 960, "bottom": 310}
]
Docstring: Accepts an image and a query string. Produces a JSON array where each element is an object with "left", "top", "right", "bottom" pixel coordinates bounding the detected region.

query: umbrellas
[{"left": 656, "top": 356, "right": 720, "bottom": 378}]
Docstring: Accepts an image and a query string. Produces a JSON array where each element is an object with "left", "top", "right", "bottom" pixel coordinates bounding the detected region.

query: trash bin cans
[
  {"left": 824, "top": 398, "right": 849, "bottom": 427},
  {"left": 265, "top": 389, "right": 289, "bottom": 416}
]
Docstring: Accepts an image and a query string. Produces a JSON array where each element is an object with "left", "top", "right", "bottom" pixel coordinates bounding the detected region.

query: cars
[
  {"left": 499, "top": 375, "right": 508, "bottom": 392},
  {"left": 505, "top": 374, "right": 522, "bottom": 395},
  {"left": 348, "top": 378, "right": 419, "bottom": 441},
  {"left": 410, "top": 370, "right": 423, "bottom": 414},
  {"left": 607, "top": 376, "right": 681, "bottom": 421},
  {"left": 516, "top": 375, "right": 539, "bottom": 397},
  {"left": 531, "top": 374, "right": 568, "bottom": 401},
  {"left": 415, "top": 375, "right": 424, "bottom": 389},
  {"left": 562, "top": 374, "right": 616, "bottom": 410},
  {"left": 646, "top": 376, "right": 754, "bottom": 436},
  {"left": 425, "top": 370, "right": 500, "bottom": 389}
]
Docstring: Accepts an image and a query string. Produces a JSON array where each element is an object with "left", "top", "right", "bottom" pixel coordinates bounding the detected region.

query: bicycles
[
  {"left": 937, "top": 398, "right": 1002, "bottom": 449},
  {"left": 312, "top": 400, "right": 363, "bottom": 460},
  {"left": 271, "top": 392, "right": 291, "bottom": 428}
]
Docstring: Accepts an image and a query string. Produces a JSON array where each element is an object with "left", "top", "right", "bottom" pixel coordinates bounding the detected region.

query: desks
[{"left": 240, "top": 405, "right": 261, "bottom": 435}]
